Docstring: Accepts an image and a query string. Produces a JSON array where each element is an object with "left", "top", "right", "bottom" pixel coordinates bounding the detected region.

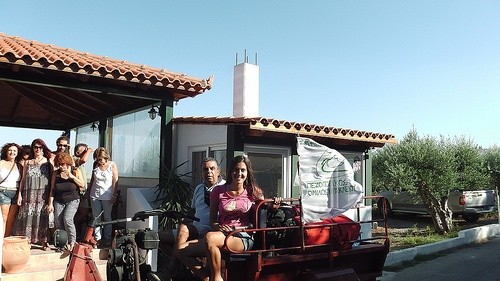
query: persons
[
  {"left": 147, "top": 157, "right": 226, "bottom": 281},
  {"left": 83, "top": 147, "right": 118, "bottom": 246},
  {"left": 0, "top": 137, "right": 94, "bottom": 251},
  {"left": 174, "top": 155, "right": 284, "bottom": 281}
]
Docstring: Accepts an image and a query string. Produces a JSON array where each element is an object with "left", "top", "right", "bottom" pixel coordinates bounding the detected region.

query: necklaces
[{"left": 33, "top": 157, "right": 43, "bottom": 166}]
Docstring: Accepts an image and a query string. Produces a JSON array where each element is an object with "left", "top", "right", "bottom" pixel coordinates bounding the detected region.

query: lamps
[
  {"left": 148, "top": 101, "right": 164, "bottom": 120},
  {"left": 90, "top": 120, "right": 101, "bottom": 132}
]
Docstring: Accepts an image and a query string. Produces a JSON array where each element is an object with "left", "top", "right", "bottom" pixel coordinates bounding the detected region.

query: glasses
[
  {"left": 34, "top": 146, "right": 44, "bottom": 149},
  {"left": 58, "top": 144, "right": 68, "bottom": 147}
]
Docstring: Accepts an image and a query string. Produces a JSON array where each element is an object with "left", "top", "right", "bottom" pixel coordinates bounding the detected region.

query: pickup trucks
[{"left": 374, "top": 176, "right": 496, "bottom": 222}]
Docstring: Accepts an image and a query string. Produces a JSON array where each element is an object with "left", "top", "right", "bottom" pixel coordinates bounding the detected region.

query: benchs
[{"left": 229, "top": 203, "right": 269, "bottom": 265}]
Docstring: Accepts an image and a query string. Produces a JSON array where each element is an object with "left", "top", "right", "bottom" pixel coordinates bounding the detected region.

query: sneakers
[{"left": 147, "top": 267, "right": 177, "bottom": 281}]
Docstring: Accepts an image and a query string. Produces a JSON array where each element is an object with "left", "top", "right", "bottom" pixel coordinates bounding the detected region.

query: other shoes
[
  {"left": 42, "top": 242, "right": 51, "bottom": 251},
  {"left": 104, "top": 240, "right": 111, "bottom": 246}
]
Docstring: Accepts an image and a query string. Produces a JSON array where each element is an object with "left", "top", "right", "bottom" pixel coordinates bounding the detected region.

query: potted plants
[{"left": 149, "top": 152, "right": 199, "bottom": 245}]
